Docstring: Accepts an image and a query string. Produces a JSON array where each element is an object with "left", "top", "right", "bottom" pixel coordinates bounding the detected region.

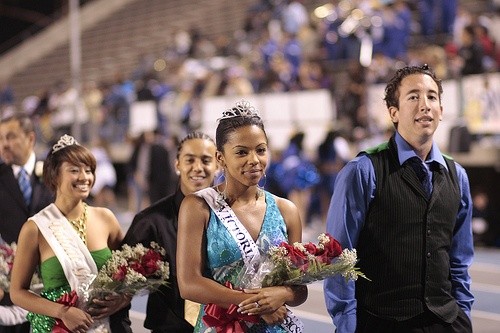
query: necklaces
[{"left": 66, "top": 202, "right": 88, "bottom": 247}]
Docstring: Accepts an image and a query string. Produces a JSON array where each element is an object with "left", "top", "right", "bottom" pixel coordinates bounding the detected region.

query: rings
[{"left": 255, "top": 301, "right": 262, "bottom": 308}]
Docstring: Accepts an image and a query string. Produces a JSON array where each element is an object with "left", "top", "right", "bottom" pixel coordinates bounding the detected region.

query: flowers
[
  {"left": 262, "top": 233, "right": 372, "bottom": 286},
  {"left": 0, "top": 238, "right": 42, "bottom": 298},
  {"left": 83, "top": 244, "right": 172, "bottom": 316}
]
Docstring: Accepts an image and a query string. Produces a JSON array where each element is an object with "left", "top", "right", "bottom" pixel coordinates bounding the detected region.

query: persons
[
  {"left": 176, "top": 100, "right": 308, "bottom": 333},
  {"left": 323, "top": 64, "right": 474, "bottom": 333},
  {"left": 0, "top": 0, "right": 500, "bottom": 248},
  {"left": 0, "top": 112, "right": 57, "bottom": 245},
  {"left": 110, "top": 131, "right": 219, "bottom": 333},
  {"left": 0, "top": 273, "right": 45, "bottom": 333},
  {"left": 10, "top": 135, "right": 124, "bottom": 333}
]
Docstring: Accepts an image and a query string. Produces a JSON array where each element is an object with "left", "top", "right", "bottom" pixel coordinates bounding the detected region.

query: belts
[{"left": 406, "top": 312, "right": 433, "bottom": 328}]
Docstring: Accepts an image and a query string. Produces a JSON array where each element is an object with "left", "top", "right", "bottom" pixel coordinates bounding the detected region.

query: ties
[
  {"left": 418, "top": 163, "right": 433, "bottom": 200},
  {"left": 17, "top": 168, "right": 32, "bottom": 208}
]
